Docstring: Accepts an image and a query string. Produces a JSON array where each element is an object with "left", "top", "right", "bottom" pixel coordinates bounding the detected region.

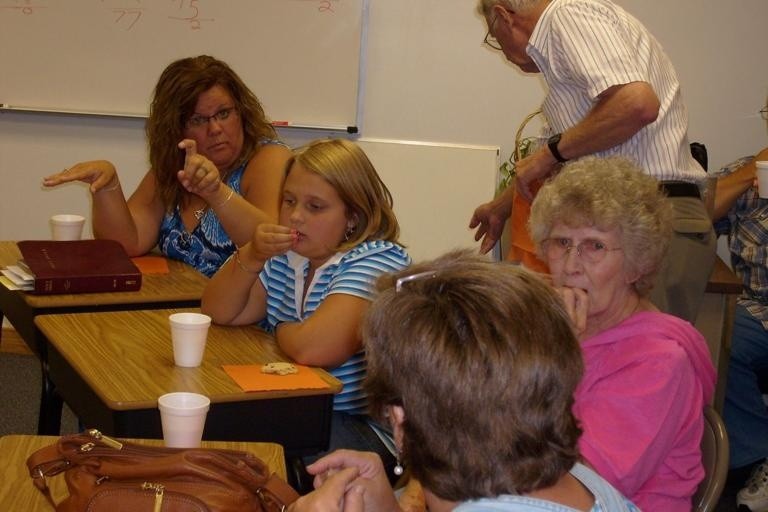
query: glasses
[
  {"left": 392, "top": 268, "right": 437, "bottom": 294},
  {"left": 540, "top": 238, "right": 622, "bottom": 262},
  {"left": 484, "top": 9, "right": 514, "bottom": 50},
  {"left": 189, "top": 107, "right": 236, "bottom": 126}
]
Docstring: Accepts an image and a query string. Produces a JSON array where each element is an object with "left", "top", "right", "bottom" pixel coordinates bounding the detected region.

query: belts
[{"left": 659, "top": 181, "right": 700, "bottom": 198}]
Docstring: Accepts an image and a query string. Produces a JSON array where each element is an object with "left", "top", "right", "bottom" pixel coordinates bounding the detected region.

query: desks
[
  {"left": 34, "top": 306, "right": 345, "bottom": 496},
  {"left": 0, "top": 239, "right": 211, "bottom": 436},
  {"left": 0, "top": 434, "right": 287, "bottom": 512},
  {"left": 699, "top": 253, "right": 745, "bottom": 421}
]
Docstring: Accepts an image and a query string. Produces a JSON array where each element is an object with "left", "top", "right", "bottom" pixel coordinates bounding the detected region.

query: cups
[
  {"left": 49, "top": 214, "right": 86, "bottom": 241},
  {"left": 755, "top": 160, "right": 768, "bottom": 199},
  {"left": 158, "top": 392, "right": 211, "bottom": 448},
  {"left": 168, "top": 312, "right": 212, "bottom": 368}
]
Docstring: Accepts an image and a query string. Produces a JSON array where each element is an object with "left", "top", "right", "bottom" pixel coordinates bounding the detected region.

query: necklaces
[
  {"left": 62, "top": 168, "right": 72, "bottom": 174},
  {"left": 189, "top": 167, "right": 229, "bottom": 220}
]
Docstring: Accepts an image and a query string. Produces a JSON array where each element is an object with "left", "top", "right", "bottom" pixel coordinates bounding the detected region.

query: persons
[
  {"left": 466, "top": 0, "right": 719, "bottom": 326},
  {"left": 698, "top": 94, "right": 767, "bottom": 511},
  {"left": 42, "top": 56, "right": 295, "bottom": 280},
  {"left": 524, "top": 153, "right": 718, "bottom": 512},
  {"left": 283, "top": 244, "right": 639, "bottom": 512},
  {"left": 199, "top": 136, "right": 416, "bottom": 504}
]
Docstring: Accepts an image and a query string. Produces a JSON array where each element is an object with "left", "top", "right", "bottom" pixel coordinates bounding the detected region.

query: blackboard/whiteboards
[
  {"left": 1, "top": 1, "right": 367, "bottom": 134},
  {"left": 1, "top": 110, "right": 501, "bottom": 331}
]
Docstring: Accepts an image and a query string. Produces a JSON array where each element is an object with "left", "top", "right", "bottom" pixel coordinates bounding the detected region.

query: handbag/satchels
[{"left": 27, "top": 429, "right": 299, "bottom": 512}]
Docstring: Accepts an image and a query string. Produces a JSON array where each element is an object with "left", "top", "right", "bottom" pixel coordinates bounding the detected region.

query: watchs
[{"left": 547, "top": 132, "right": 570, "bottom": 162}]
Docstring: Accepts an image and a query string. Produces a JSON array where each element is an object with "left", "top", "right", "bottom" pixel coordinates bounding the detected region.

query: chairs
[{"left": 688, "top": 405, "right": 731, "bottom": 512}]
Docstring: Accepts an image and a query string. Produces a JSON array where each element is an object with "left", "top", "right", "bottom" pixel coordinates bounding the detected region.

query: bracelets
[
  {"left": 88, "top": 181, "right": 120, "bottom": 192},
  {"left": 212, "top": 189, "right": 233, "bottom": 211},
  {"left": 219, "top": 243, "right": 262, "bottom": 274}
]
